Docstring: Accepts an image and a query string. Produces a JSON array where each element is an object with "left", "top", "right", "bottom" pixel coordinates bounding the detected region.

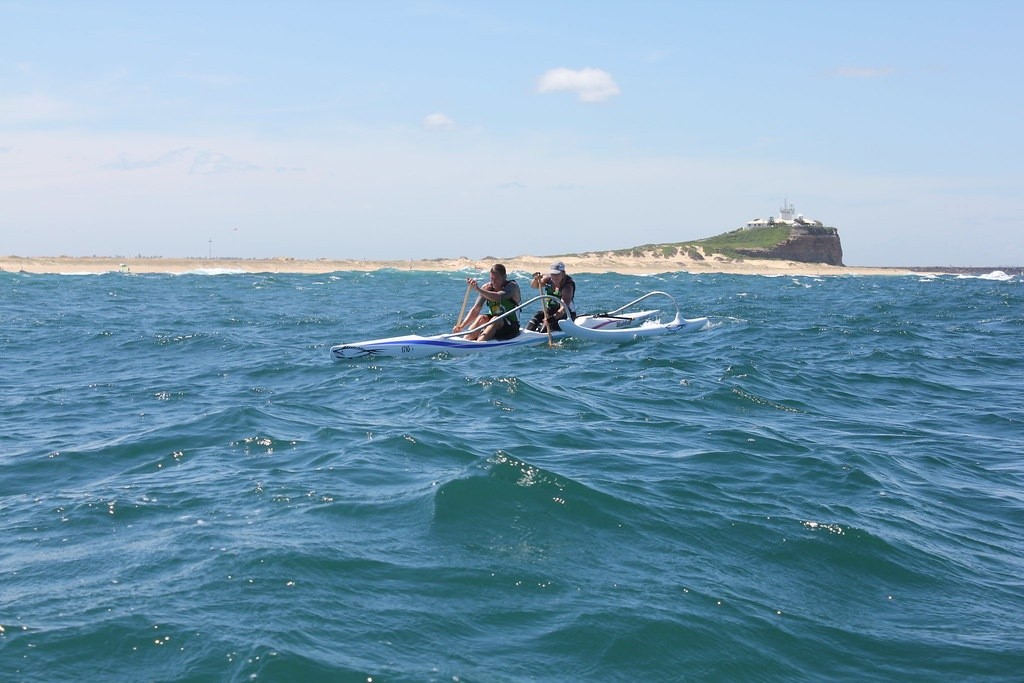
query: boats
[{"left": 328, "top": 289, "right": 710, "bottom": 363}]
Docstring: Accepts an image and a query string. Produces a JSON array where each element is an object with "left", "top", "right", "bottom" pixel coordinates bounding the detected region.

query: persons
[
  {"left": 526, "top": 262, "right": 576, "bottom": 333},
  {"left": 452, "top": 264, "right": 521, "bottom": 342}
]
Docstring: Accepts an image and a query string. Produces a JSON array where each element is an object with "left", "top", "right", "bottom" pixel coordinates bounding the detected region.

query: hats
[{"left": 548, "top": 261, "right": 565, "bottom": 274}]
